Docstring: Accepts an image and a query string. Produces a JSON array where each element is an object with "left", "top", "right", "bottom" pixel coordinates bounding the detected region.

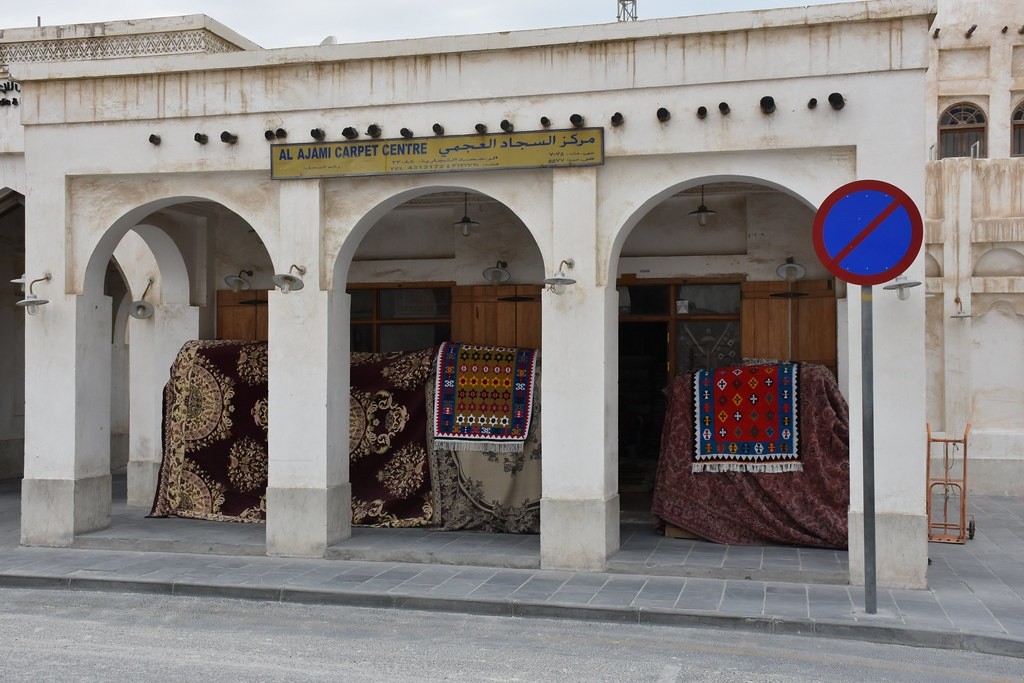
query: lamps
[
  {"left": 10, "top": 274, "right": 25, "bottom": 293},
  {"left": 484, "top": 260, "right": 511, "bottom": 286},
  {"left": 689, "top": 186, "right": 717, "bottom": 226},
  {"left": 453, "top": 193, "right": 480, "bottom": 236},
  {"left": 272, "top": 265, "right": 307, "bottom": 294},
  {"left": 542, "top": 258, "right": 577, "bottom": 295},
  {"left": 776, "top": 257, "right": 805, "bottom": 284},
  {"left": 128, "top": 281, "right": 153, "bottom": 320},
  {"left": 14, "top": 271, "right": 53, "bottom": 315},
  {"left": 224, "top": 270, "right": 254, "bottom": 293},
  {"left": 881, "top": 274, "right": 922, "bottom": 301},
  {"left": 950, "top": 297, "right": 972, "bottom": 324}
]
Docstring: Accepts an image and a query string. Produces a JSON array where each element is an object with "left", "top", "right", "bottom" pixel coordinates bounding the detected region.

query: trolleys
[{"left": 926, "top": 422, "right": 982, "bottom": 540}]
